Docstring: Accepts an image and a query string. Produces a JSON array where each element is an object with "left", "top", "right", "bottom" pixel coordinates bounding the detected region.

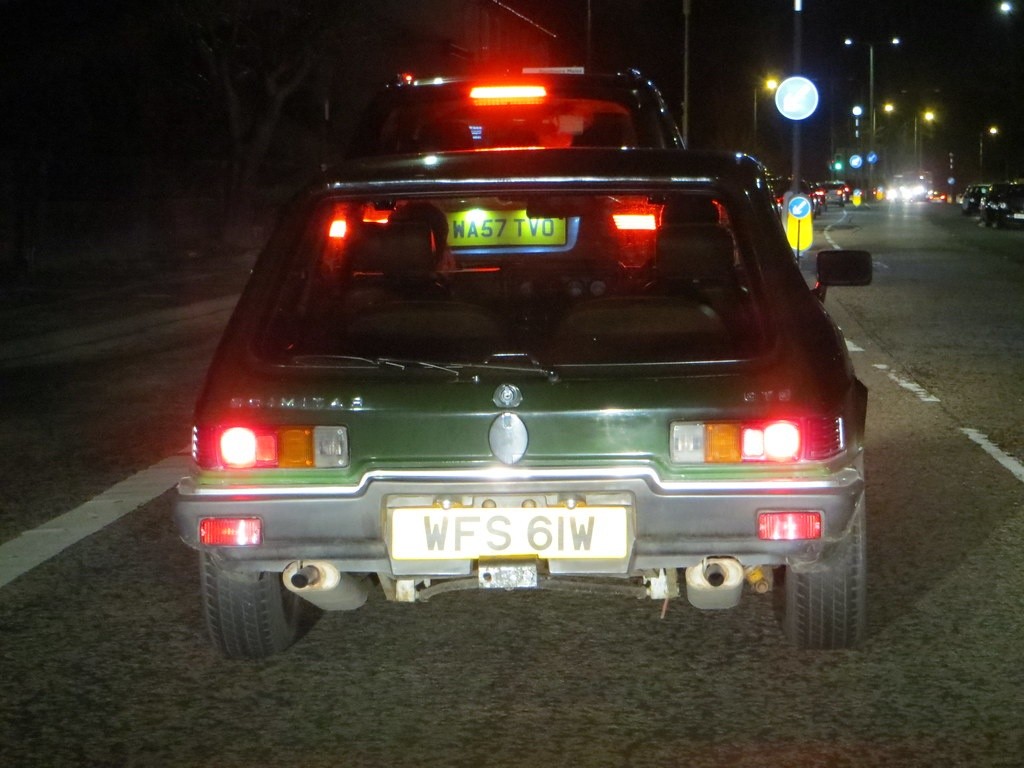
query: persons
[
  {"left": 387, "top": 201, "right": 495, "bottom": 304},
  {"left": 635, "top": 198, "right": 719, "bottom": 293}
]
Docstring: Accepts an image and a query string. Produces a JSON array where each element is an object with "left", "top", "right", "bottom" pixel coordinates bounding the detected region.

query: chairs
[
  {"left": 360, "top": 220, "right": 437, "bottom": 300},
  {"left": 556, "top": 294, "right": 732, "bottom": 340},
  {"left": 654, "top": 223, "right": 756, "bottom": 338},
  {"left": 345, "top": 299, "right": 520, "bottom": 351}
]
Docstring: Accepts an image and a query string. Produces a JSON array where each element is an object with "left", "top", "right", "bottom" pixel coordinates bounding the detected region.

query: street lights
[
  {"left": 753, "top": 79, "right": 777, "bottom": 150},
  {"left": 914, "top": 110, "right": 934, "bottom": 169},
  {"left": 844, "top": 37, "right": 900, "bottom": 204},
  {"left": 980, "top": 126, "right": 998, "bottom": 183}
]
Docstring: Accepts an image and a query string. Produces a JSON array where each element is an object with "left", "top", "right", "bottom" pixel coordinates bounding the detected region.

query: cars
[
  {"left": 173, "top": 146, "right": 874, "bottom": 660},
  {"left": 885, "top": 172, "right": 1024, "bottom": 229},
  {"left": 768, "top": 167, "right": 887, "bottom": 218}
]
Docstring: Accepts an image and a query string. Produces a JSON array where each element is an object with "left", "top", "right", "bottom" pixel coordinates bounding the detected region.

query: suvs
[{"left": 357, "top": 66, "right": 686, "bottom": 267}]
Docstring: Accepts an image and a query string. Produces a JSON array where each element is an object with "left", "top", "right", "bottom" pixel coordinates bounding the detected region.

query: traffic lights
[{"left": 833, "top": 154, "right": 843, "bottom": 170}]
